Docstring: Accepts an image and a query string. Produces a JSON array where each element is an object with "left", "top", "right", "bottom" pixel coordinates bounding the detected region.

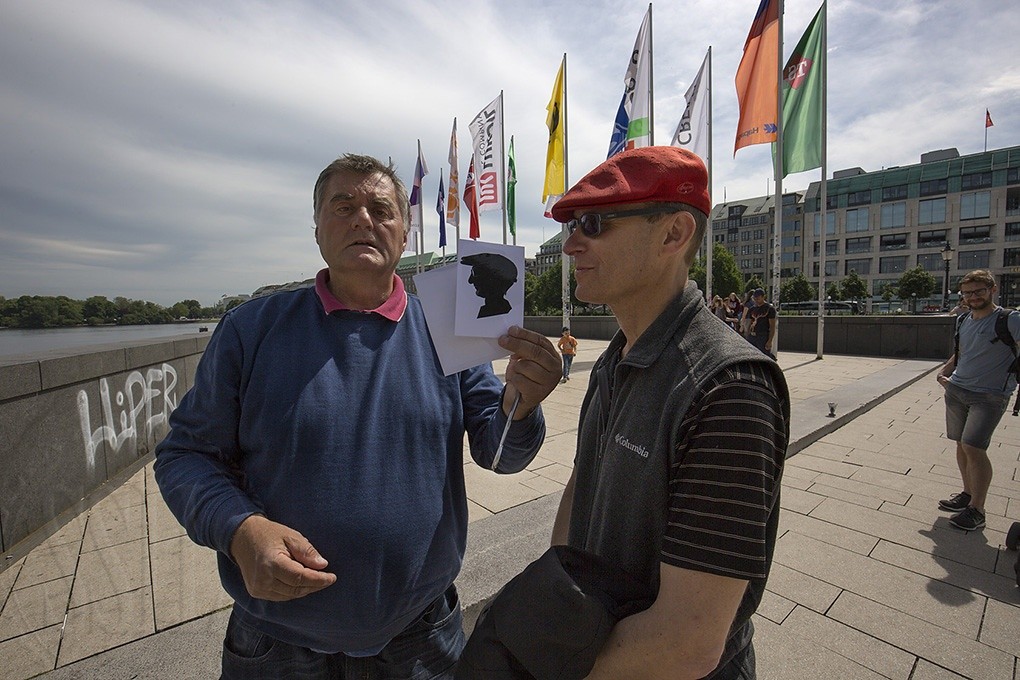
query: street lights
[
  {"left": 940, "top": 239, "right": 956, "bottom": 313},
  {"left": 826, "top": 296, "right": 831, "bottom": 316},
  {"left": 911, "top": 293, "right": 916, "bottom": 315}
]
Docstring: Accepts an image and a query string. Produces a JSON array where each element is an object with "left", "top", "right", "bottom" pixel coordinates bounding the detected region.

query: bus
[
  {"left": 837, "top": 301, "right": 863, "bottom": 315},
  {"left": 780, "top": 301, "right": 854, "bottom": 316}
]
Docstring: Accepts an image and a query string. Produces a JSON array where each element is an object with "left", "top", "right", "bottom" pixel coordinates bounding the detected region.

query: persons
[
  {"left": 551, "top": 145, "right": 790, "bottom": 680},
  {"left": 708, "top": 293, "right": 741, "bottom": 331},
  {"left": 937, "top": 269, "right": 1020, "bottom": 532},
  {"left": 559, "top": 327, "right": 578, "bottom": 383},
  {"left": 738, "top": 288, "right": 778, "bottom": 362},
  {"left": 151, "top": 156, "right": 563, "bottom": 680}
]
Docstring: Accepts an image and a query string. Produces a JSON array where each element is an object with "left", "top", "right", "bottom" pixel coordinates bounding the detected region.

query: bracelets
[{"left": 937, "top": 375, "right": 943, "bottom": 381}]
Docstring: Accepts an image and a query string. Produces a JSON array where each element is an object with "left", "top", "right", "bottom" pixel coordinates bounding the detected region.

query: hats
[{"left": 551, "top": 147, "right": 712, "bottom": 224}]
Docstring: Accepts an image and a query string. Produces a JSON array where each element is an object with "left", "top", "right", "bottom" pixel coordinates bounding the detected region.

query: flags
[
  {"left": 463, "top": 156, "right": 480, "bottom": 241},
  {"left": 542, "top": 61, "right": 565, "bottom": 218},
  {"left": 436, "top": 173, "right": 447, "bottom": 248},
  {"left": 409, "top": 143, "right": 429, "bottom": 231},
  {"left": 402, "top": 230, "right": 416, "bottom": 251},
  {"left": 734, "top": 0, "right": 779, "bottom": 157},
  {"left": 770, "top": 4, "right": 823, "bottom": 180},
  {"left": 606, "top": 9, "right": 651, "bottom": 162},
  {"left": 986, "top": 111, "right": 994, "bottom": 128},
  {"left": 468, "top": 94, "right": 504, "bottom": 215},
  {"left": 506, "top": 138, "right": 517, "bottom": 246},
  {"left": 446, "top": 119, "right": 460, "bottom": 228},
  {"left": 670, "top": 51, "right": 708, "bottom": 169}
]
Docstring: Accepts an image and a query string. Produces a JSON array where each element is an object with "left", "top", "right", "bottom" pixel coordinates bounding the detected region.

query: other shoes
[
  {"left": 562, "top": 378, "right": 567, "bottom": 383},
  {"left": 567, "top": 376, "right": 570, "bottom": 380}
]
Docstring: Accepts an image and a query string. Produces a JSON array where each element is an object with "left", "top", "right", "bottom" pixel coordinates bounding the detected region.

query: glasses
[
  {"left": 565, "top": 207, "right": 682, "bottom": 239},
  {"left": 961, "top": 284, "right": 995, "bottom": 298}
]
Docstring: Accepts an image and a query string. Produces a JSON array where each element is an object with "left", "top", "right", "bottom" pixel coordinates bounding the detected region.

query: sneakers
[
  {"left": 949, "top": 507, "right": 987, "bottom": 531},
  {"left": 937, "top": 491, "right": 971, "bottom": 511}
]
